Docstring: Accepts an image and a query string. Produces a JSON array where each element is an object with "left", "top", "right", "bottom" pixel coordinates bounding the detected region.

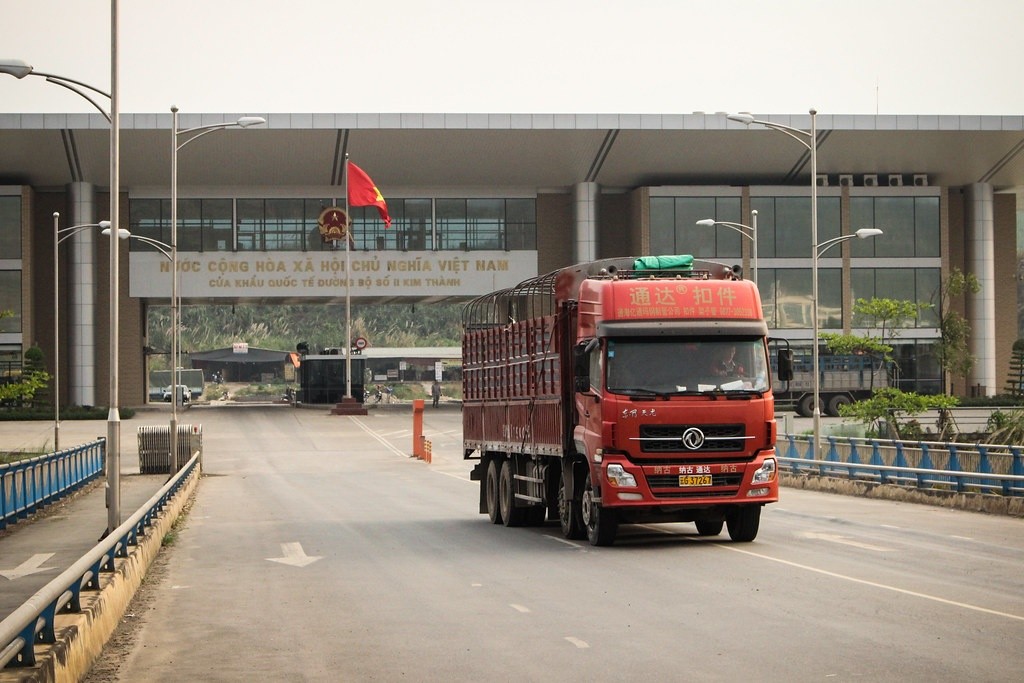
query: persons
[
  {"left": 286, "top": 386, "right": 294, "bottom": 402},
  {"left": 364, "top": 385, "right": 393, "bottom": 404},
  {"left": 430, "top": 380, "right": 442, "bottom": 408},
  {"left": 710, "top": 343, "right": 744, "bottom": 378}
]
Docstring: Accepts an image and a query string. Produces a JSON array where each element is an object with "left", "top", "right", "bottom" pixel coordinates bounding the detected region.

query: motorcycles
[{"left": 374, "top": 391, "right": 382, "bottom": 404}]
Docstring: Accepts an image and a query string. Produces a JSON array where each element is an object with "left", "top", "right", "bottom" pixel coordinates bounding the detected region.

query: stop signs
[{"left": 355, "top": 337, "right": 367, "bottom": 349}]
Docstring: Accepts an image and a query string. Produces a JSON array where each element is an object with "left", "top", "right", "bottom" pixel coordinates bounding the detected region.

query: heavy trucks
[
  {"left": 769, "top": 355, "right": 889, "bottom": 417},
  {"left": 460, "top": 255, "right": 779, "bottom": 547}
]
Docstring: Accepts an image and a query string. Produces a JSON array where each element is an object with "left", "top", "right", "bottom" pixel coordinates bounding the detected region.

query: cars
[{"left": 163, "top": 385, "right": 192, "bottom": 402}]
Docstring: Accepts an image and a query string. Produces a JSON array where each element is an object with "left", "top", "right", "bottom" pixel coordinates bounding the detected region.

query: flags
[{"left": 346, "top": 160, "right": 392, "bottom": 229}]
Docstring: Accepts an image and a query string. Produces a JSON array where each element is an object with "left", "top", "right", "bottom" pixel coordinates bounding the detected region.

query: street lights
[
  {"left": 696, "top": 210, "right": 758, "bottom": 286},
  {"left": 53, "top": 211, "right": 110, "bottom": 453},
  {"left": 0, "top": 0, "right": 122, "bottom": 537},
  {"left": 725, "top": 108, "right": 884, "bottom": 460},
  {"left": 100, "top": 106, "right": 266, "bottom": 480}
]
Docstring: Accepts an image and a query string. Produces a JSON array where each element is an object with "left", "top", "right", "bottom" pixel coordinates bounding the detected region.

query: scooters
[
  {"left": 211, "top": 374, "right": 225, "bottom": 383},
  {"left": 279, "top": 388, "right": 297, "bottom": 403}
]
